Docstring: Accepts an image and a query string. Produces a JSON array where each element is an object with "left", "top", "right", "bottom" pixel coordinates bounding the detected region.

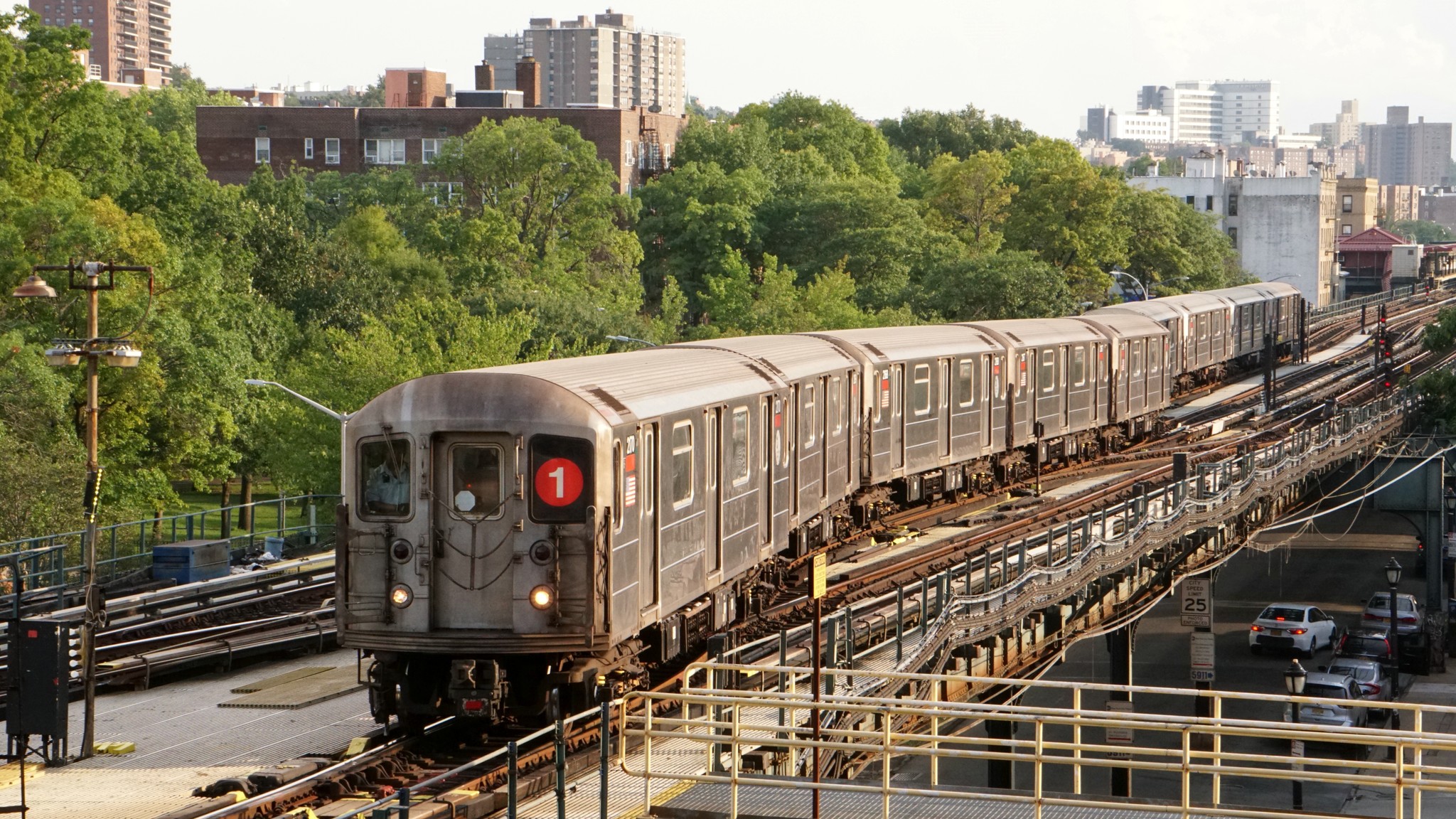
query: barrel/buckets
[{"left": 264, "top": 536, "right": 285, "bottom": 560}]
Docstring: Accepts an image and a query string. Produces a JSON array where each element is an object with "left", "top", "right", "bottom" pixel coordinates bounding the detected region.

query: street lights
[
  {"left": 12, "top": 256, "right": 153, "bottom": 676},
  {"left": 1109, "top": 271, "right": 1189, "bottom": 301},
  {"left": 243, "top": 379, "right": 358, "bottom": 507},
  {"left": 1383, "top": 558, "right": 1403, "bottom": 761},
  {"left": 1283, "top": 658, "right": 1308, "bottom": 812},
  {"left": 1444, "top": 487, "right": 1456, "bottom": 598}
]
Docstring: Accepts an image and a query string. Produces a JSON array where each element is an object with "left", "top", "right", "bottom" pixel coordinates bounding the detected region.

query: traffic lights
[
  {"left": 1380, "top": 306, "right": 1386, "bottom": 356},
  {"left": 1384, "top": 335, "right": 1394, "bottom": 395},
  {"left": 1426, "top": 284, "right": 1429, "bottom": 300},
  {"left": 1417, "top": 536, "right": 1425, "bottom": 578}
]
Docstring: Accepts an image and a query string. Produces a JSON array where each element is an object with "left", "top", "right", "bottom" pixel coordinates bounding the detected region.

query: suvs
[
  {"left": 1328, "top": 624, "right": 1431, "bottom": 685},
  {"left": 1283, "top": 673, "right": 1370, "bottom": 752}
]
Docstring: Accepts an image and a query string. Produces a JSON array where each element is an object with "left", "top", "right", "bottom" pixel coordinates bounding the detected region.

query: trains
[{"left": 334, "top": 281, "right": 1302, "bottom": 748}]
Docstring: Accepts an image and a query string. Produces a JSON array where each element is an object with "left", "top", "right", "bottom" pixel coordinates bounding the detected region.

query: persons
[{"left": 366, "top": 439, "right": 411, "bottom": 516}]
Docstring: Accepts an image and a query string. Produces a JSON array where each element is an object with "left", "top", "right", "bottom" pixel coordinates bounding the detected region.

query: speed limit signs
[{"left": 1180, "top": 578, "right": 1210, "bottom": 615}]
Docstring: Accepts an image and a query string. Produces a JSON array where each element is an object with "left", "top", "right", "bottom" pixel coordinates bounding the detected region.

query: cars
[
  {"left": 1359, "top": 590, "right": 1426, "bottom": 634},
  {"left": 1325, "top": 658, "right": 1393, "bottom": 718},
  {"left": 1249, "top": 602, "right": 1337, "bottom": 660}
]
